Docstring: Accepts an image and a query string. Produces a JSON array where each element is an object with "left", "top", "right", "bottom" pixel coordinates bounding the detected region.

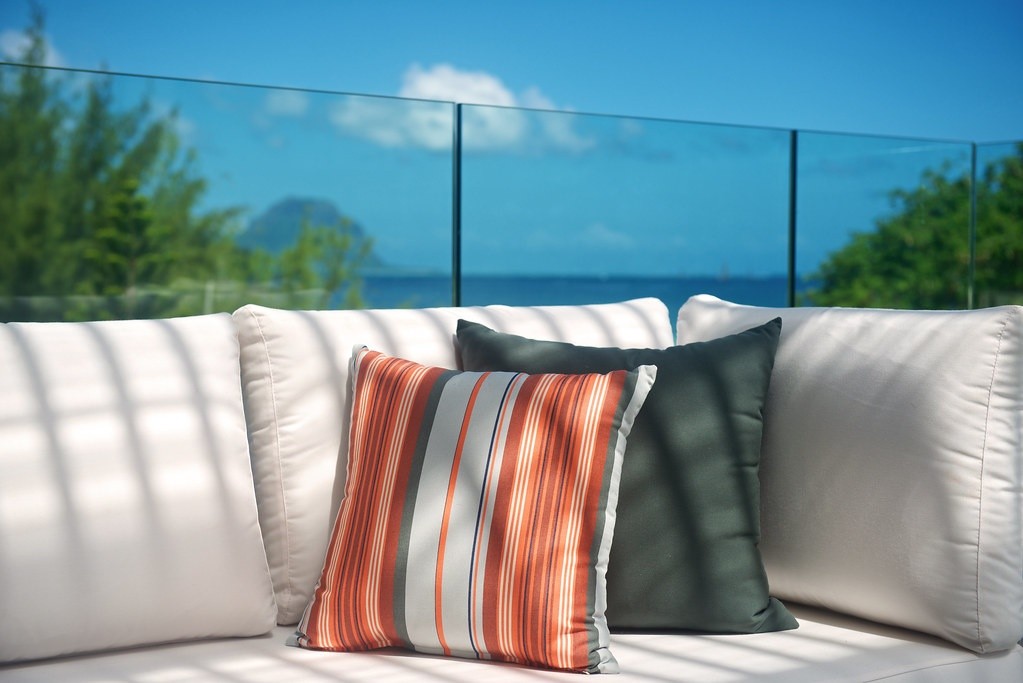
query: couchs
[{"left": 0, "top": 291, "right": 1023, "bottom": 683}]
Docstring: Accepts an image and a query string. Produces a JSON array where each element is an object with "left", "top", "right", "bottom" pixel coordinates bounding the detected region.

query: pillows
[
  {"left": 455, "top": 316, "right": 799, "bottom": 635},
  {"left": 295, "top": 345, "right": 658, "bottom": 674}
]
